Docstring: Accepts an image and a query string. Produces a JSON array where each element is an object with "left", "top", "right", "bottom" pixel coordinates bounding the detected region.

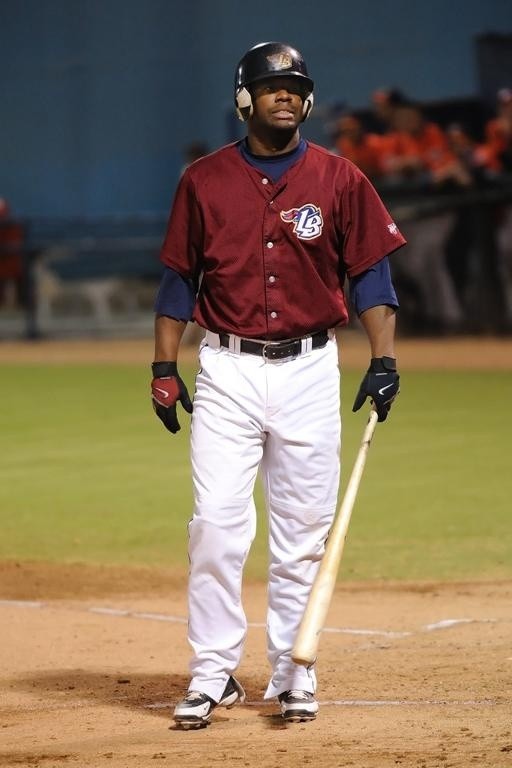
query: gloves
[
  {"left": 151, "top": 362, "right": 193, "bottom": 434},
  {"left": 352, "top": 356, "right": 401, "bottom": 422}
]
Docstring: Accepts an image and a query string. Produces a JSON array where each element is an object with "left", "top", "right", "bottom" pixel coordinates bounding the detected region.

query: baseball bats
[{"left": 291, "top": 403, "right": 378, "bottom": 665}]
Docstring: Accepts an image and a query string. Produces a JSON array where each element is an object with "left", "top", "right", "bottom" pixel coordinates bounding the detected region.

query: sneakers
[
  {"left": 172, "top": 676, "right": 245, "bottom": 729},
  {"left": 278, "top": 689, "right": 320, "bottom": 723}
]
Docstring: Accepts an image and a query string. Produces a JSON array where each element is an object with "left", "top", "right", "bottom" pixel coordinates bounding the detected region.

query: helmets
[{"left": 235, "top": 41, "right": 314, "bottom": 122}]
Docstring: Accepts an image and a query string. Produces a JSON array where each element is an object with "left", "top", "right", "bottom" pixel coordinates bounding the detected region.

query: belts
[{"left": 219, "top": 333, "right": 329, "bottom": 359}]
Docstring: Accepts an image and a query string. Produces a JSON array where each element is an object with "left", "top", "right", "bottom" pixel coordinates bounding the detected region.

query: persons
[
  {"left": 151, "top": 39, "right": 407, "bottom": 729},
  {"left": 178, "top": 83, "right": 510, "bottom": 328}
]
